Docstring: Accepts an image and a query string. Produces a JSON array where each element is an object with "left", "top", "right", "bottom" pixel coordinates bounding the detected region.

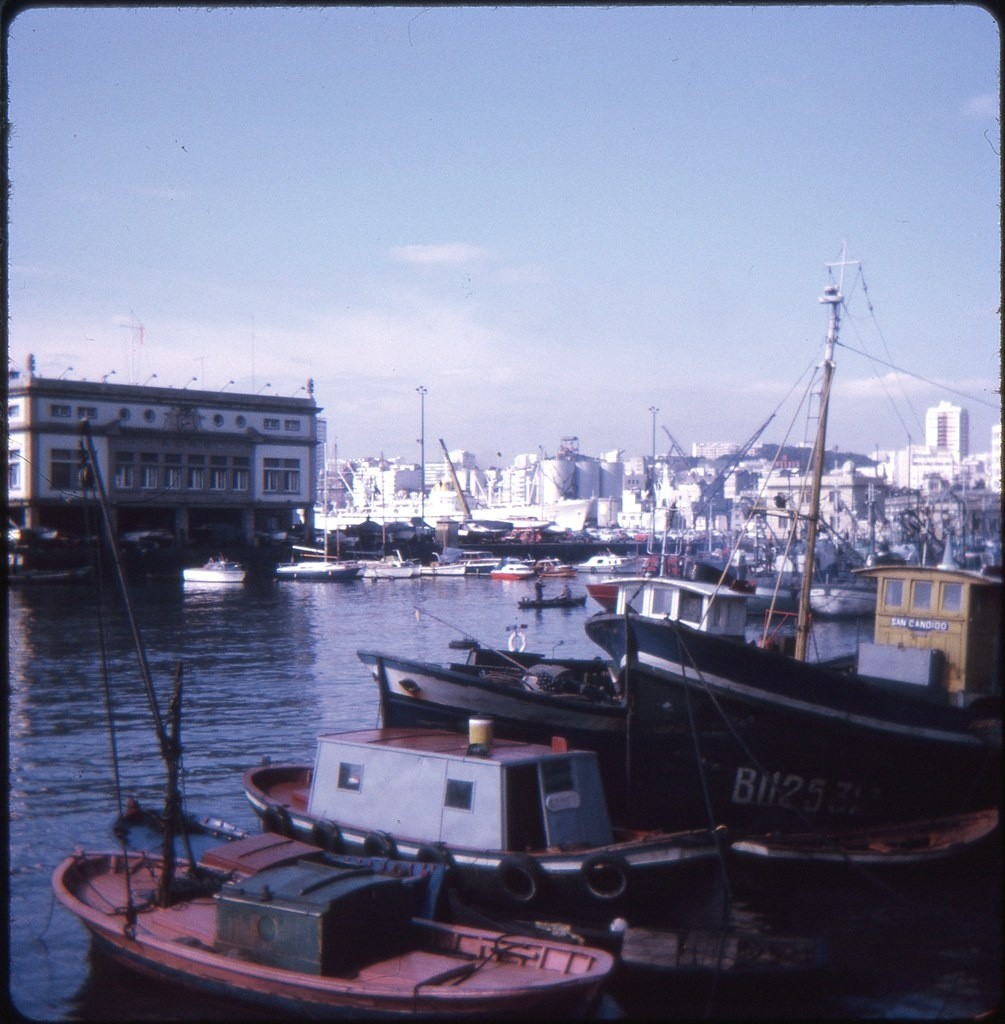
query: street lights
[
  {"left": 416, "top": 385, "right": 429, "bottom": 545},
  {"left": 646, "top": 407, "right": 661, "bottom": 536}
]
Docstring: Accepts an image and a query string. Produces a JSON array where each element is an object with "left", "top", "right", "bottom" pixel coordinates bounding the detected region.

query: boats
[
  {"left": 578, "top": 548, "right": 646, "bottom": 575},
  {"left": 580, "top": 245, "right": 1005, "bottom": 816},
  {"left": 180, "top": 551, "right": 247, "bottom": 585},
  {"left": 354, "top": 635, "right": 623, "bottom": 749},
  {"left": 457, "top": 549, "right": 502, "bottom": 576},
  {"left": 490, "top": 564, "right": 536, "bottom": 581},
  {"left": 415, "top": 563, "right": 468, "bottom": 578},
  {"left": 352, "top": 556, "right": 422, "bottom": 579},
  {"left": 533, "top": 557, "right": 579, "bottom": 580},
  {"left": 50, "top": 414, "right": 618, "bottom": 1024},
  {"left": 273, "top": 560, "right": 363, "bottom": 581},
  {"left": 241, "top": 716, "right": 730, "bottom": 919},
  {"left": 726, "top": 808, "right": 1005, "bottom": 897}
]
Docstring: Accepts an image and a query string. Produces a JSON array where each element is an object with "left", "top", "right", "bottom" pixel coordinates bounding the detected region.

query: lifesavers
[
  {"left": 307, "top": 816, "right": 346, "bottom": 856},
  {"left": 413, "top": 843, "right": 460, "bottom": 884},
  {"left": 508, "top": 631, "right": 527, "bottom": 652},
  {"left": 361, "top": 829, "right": 398, "bottom": 862},
  {"left": 493, "top": 851, "right": 547, "bottom": 908},
  {"left": 577, "top": 850, "right": 634, "bottom": 906},
  {"left": 261, "top": 804, "right": 294, "bottom": 839}
]
